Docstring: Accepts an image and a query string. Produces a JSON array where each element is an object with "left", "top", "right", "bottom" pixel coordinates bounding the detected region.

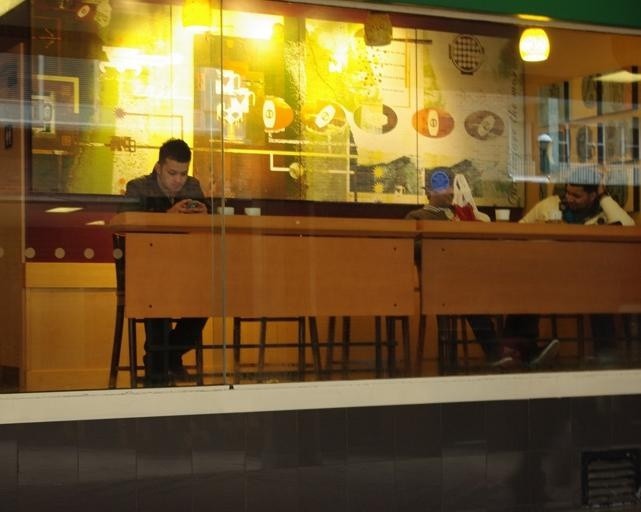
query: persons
[
  {"left": 404, "top": 166, "right": 560, "bottom": 370},
  {"left": 117, "top": 139, "right": 211, "bottom": 385},
  {"left": 518, "top": 165, "right": 636, "bottom": 365}
]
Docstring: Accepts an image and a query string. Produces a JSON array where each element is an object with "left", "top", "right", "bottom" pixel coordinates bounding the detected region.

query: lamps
[
  {"left": 518, "top": 25, "right": 551, "bottom": 63},
  {"left": 364, "top": 9, "right": 394, "bottom": 45}
]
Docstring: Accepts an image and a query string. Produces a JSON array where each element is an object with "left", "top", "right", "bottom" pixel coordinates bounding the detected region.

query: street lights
[{"left": 538, "top": 134, "right": 551, "bottom": 174}]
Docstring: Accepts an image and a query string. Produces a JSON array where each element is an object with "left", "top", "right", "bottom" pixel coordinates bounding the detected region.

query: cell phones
[{"left": 188, "top": 200, "right": 198, "bottom": 208}]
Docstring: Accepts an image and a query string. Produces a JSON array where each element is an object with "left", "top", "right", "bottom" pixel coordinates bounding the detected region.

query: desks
[{"left": 104, "top": 209, "right": 640, "bottom": 376}]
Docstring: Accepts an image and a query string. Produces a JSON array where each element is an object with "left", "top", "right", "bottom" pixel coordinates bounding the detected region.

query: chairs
[{"left": 109, "top": 194, "right": 203, "bottom": 389}]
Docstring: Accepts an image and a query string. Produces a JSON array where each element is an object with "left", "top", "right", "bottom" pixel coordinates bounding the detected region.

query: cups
[
  {"left": 244, "top": 208, "right": 261, "bottom": 217},
  {"left": 495, "top": 208, "right": 510, "bottom": 221},
  {"left": 216, "top": 206, "right": 235, "bottom": 215}
]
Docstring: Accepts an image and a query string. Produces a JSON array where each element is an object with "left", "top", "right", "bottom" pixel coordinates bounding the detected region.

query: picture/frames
[{"left": 32, "top": 73, "right": 80, "bottom": 157}]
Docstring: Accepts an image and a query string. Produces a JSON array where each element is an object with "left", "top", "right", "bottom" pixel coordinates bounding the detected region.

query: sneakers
[
  {"left": 146, "top": 354, "right": 186, "bottom": 384},
  {"left": 490, "top": 339, "right": 559, "bottom": 367}
]
